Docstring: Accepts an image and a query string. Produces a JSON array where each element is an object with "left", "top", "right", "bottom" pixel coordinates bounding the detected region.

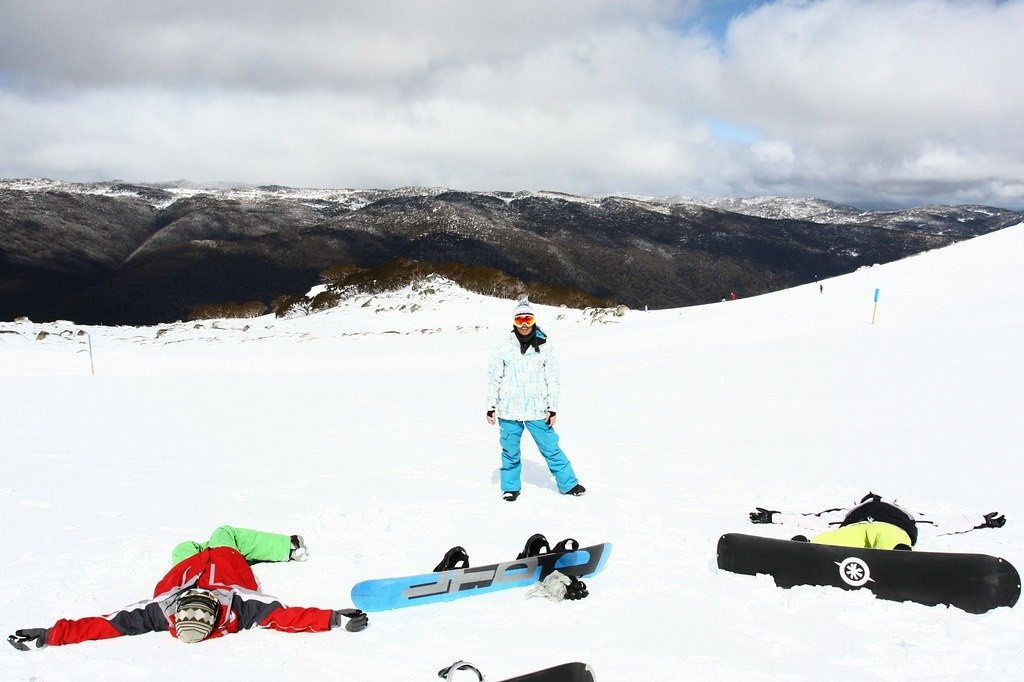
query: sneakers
[
  {"left": 504, "top": 491, "right": 519, "bottom": 501},
  {"left": 566, "top": 484, "right": 585, "bottom": 496},
  {"left": 290, "top": 535, "right": 307, "bottom": 562}
]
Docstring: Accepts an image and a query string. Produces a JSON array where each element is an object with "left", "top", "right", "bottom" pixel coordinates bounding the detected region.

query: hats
[
  {"left": 512, "top": 299, "right": 536, "bottom": 317},
  {"left": 175, "top": 589, "right": 220, "bottom": 643}
]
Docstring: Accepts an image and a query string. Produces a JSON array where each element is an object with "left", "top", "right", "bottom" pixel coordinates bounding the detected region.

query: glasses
[
  {"left": 186, "top": 590, "right": 215, "bottom": 600},
  {"left": 514, "top": 315, "right": 535, "bottom": 328}
]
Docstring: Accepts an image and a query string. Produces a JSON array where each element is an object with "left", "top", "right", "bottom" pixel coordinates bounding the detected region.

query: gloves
[
  {"left": 564, "top": 575, "right": 588, "bottom": 600},
  {"left": 545, "top": 411, "right": 557, "bottom": 430},
  {"left": 749, "top": 507, "right": 781, "bottom": 524},
  {"left": 330, "top": 609, "right": 368, "bottom": 632},
  {"left": 974, "top": 512, "right": 1006, "bottom": 529},
  {"left": 486, "top": 410, "right": 497, "bottom": 425},
  {"left": 7, "top": 628, "right": 51, "bottom": 651}
]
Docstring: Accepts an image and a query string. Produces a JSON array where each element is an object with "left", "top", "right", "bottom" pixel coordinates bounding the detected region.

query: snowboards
[
  {"left": 718, "top": 533, "right": 1021, "bottom": 614},
  {"left": 437, "top": 660, "right": 597, "bottom": 682},
  {"left": 350, "top": 534, "right": 613, "bottom": 613}
]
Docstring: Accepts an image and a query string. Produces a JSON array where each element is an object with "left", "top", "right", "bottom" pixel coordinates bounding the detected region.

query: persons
[
  {"left": 720, "top": 292, "right": 736, "bottom": 303},
  {"left": 749, "top": 493, "right": 1007, "bottom": 551},
  {"left": 814, "top": 273, "right": 824, "bottom": 293},
  {"left": 7, "top": 525, "right": 369, "bottom": 652},
  {"left": 486, "top": 294, "right": 586, "bottom": 502}
]
